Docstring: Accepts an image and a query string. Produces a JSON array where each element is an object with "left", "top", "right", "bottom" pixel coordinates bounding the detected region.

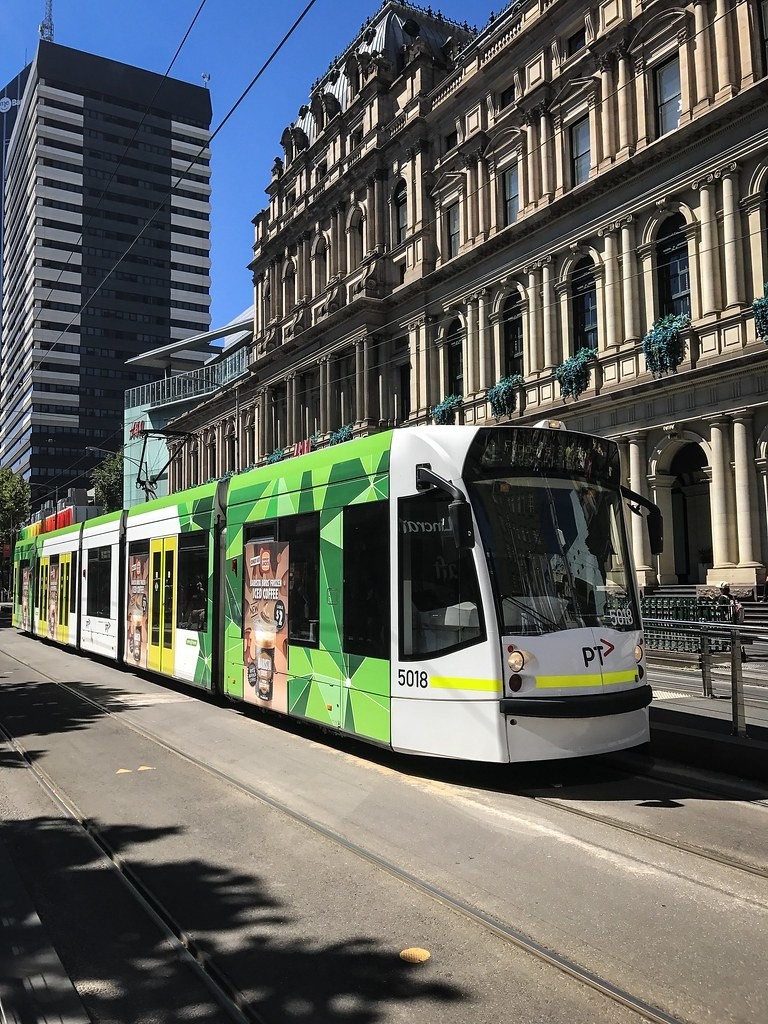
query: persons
[
  {"left": 715, "top": 582, "right": 733, "bottom": 651},
  {"left": 186, "top": 582, "right": 206, "bottom": 629}
]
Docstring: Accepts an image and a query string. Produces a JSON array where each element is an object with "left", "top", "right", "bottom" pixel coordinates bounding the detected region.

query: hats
[{"left": 715, "top": 581, "right": 730, "bottom": 589}]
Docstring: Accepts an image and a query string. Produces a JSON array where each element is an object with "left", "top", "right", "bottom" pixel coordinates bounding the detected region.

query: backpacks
[{"left": 721, "top": 594, "right": 744, "bottom": 625}]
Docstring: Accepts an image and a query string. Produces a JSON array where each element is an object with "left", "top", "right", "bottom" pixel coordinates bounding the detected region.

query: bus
[{"left": 11, "top": 424, "right": 665, "bottom": 766}]
